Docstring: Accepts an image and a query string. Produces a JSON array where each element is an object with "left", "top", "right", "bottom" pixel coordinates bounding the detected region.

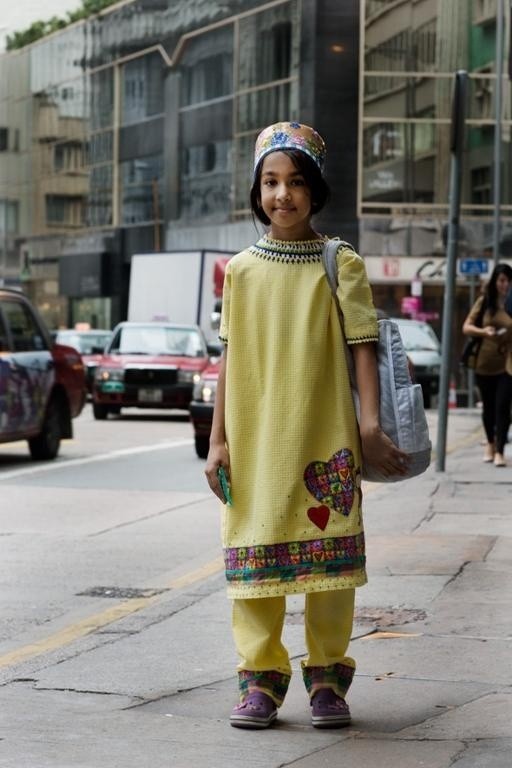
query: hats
[{"left": 251, "top": 122, "right": 327, "bottom": 177}]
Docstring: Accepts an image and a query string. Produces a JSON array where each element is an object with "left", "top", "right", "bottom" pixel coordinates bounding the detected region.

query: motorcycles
[{"left": 385, "top": 317, "right": 442, "bottom": 408}]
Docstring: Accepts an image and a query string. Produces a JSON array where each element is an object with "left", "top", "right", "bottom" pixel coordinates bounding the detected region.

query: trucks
[{"left": 126, "top": 248, "right": 238, "bottom": 353}]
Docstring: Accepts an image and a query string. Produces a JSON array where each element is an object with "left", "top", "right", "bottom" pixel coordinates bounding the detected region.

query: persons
[
  {"left": 206, "top": 121, "right": 413, "bottom": 728},
  {"left": 461, "top": 263, "right": 512, "bottom": 467}
]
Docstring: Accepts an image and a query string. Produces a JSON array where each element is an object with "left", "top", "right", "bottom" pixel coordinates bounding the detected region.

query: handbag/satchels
[
  {"left": 321, "top": 239, "right": 431, "bottom": 484},
  {"left": 462, "top": 294, "right": 491, "bottom": 368}
]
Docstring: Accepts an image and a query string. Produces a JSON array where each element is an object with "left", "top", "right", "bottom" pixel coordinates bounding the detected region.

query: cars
[{"left": 0, "top": 285, "right": 223, "bottom": 459}]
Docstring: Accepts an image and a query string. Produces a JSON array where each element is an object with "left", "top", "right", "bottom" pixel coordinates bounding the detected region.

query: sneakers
[
  {"left": 310, "top": 687, "right": 352, "bottom": 728},
  {"left": 229, "top": 692, "right": 278, "bottom": 731}
]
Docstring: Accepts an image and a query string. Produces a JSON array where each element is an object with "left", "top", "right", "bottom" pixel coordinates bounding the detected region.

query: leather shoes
[
  {"left": 495, "top": 459, "right": 506, "bottom": 466},
  {"left": 484, "top": 455, "right": 493, "bottom": 462}
]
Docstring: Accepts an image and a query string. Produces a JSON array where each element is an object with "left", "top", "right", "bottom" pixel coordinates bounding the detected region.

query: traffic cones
[{"left": 448, "top": 376, "right": 457, "bottom": 408}]
